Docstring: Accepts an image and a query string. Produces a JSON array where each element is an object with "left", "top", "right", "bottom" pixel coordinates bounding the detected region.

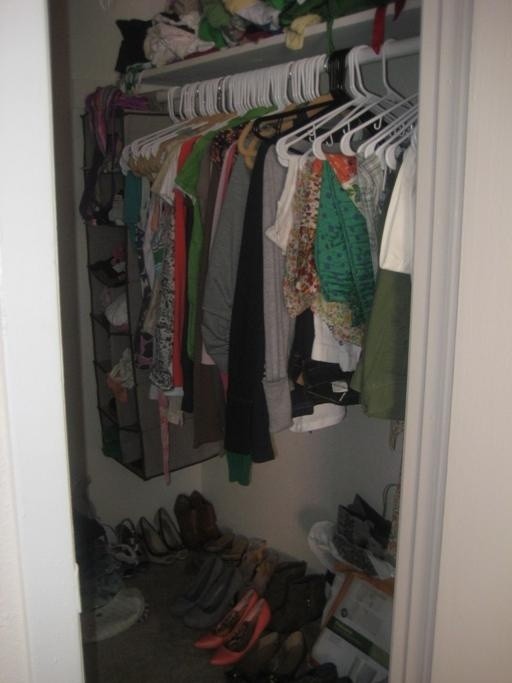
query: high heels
[
  {"left": 191, "top": 490, "right": 222, "bottom": 540},
  {"left": 192, "top": 588, "right": 258, "bottom": 649},
  {"left": 293, "top": 662, "right": 338, "bottom": 682},
  {"left": 256, "top": 630, "right": 308, "bottom": 682},
  {"left": 184, "top": 565, "right": 243, "bottom": 628},
  {"left": 135, "top": 515, "right": 176, "bottom": 564},
  {"left": 172, "top": 493, "right": 204, "bottom": 551},
  {"left": 175, "top": 555, "right": 224, "bottom": 616},
  {"left": 116, "top": 518, "right": 150, "bottom": 567},
  {"left": 224, "top": 631, "right": 280, "bottom": 682},
  {"left": 154, "top": 507, "right": 189, "bottom": 560},
  {"left": 208, "top": 597, "right": 272, "bottom": 666}
]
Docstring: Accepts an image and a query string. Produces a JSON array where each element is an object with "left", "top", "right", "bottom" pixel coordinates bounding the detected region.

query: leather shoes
[
  {"left": 222, "top": 535, "right": 248, "bottom": 559},
  {"left": 204, "top": 532, "right": 235, "bottom": 551}
]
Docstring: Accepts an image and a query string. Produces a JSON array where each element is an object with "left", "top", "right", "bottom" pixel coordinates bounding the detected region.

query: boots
[
  {"left": 263, "top": 559, "right": 307, "bottom": 613},
  {"left": 267, "top": 575, "right": 326, "bottom": 631}
]
